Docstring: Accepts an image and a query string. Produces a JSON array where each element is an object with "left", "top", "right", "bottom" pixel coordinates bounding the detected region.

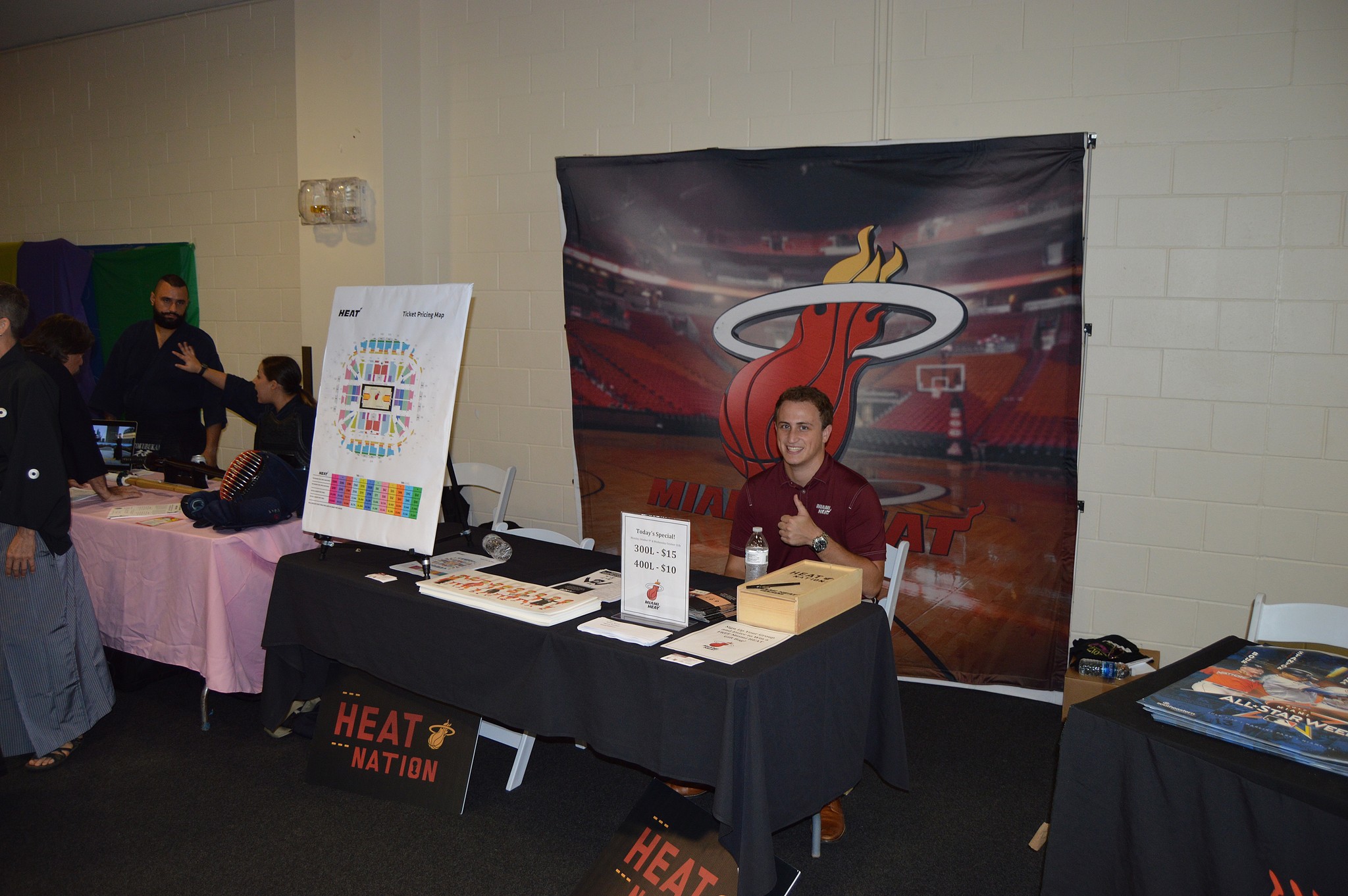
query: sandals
[{"left": 25, "top": 735, "right": 84, "bottom": 770}]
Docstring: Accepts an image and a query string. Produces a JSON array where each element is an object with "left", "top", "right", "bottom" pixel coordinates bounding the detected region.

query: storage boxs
[
  {"left": 737, "top": 559, "right": 863, "bottom": 636},
  {"left": 1062, "top": 649, "right": 1159, "bottom": 723}
]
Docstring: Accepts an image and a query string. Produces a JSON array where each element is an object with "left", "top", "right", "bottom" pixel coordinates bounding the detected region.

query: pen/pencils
[{"left": 746, "top": 582, "right": 800, "bottom": 589}]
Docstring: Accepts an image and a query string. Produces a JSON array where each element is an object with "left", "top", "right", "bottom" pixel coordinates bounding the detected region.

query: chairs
[
  {"left": 1247, "top": 592, "right": 1348, "bottom": 650},
  {"left": 865, "top": 542, "right": 909, "bottom": 634},
  {"left": 441, "top": 462, "right": 518, "bottom": 529},
  {"left": 478, "top": 523, "right": 596, "bottom": 791}
]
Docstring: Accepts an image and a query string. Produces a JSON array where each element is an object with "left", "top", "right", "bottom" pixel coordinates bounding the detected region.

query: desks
[
  {"left": 1039, "top": 635, "right": 1348, "bottom": 896},
  {"left": 70, "top": 468, "right": 342, "bottom": 731},
  {"left": 261, "top": 519, "right": 911, "bottom": 896}
]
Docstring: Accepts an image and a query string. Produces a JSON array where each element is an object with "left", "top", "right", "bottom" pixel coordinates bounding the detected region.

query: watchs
[
  {"left": 198, "top": 363, "right": 208, "bottom": 376},
  {"left": 809, "top": 533, "right": 830, "bottom": 555}
]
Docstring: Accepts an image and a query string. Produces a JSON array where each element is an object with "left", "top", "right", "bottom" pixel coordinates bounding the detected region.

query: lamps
[{"left": 298, "top": 178, "right": 368, "bottom": 225}]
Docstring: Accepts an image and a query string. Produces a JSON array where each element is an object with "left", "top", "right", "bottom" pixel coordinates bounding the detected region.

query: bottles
[
  {"left": 482, "top": 533, "right": 512, "bottom": 561},
  {"left": 1078, "top": 658, "right": 1136, "bottom": 680},
  {"left": 744, "top": 527, "right": 769, "bottom": 583}
]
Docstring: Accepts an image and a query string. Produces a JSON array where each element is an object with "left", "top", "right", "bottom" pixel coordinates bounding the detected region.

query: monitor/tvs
[{"left": 92, "top": 419, "right": 138, "bottom": 473}]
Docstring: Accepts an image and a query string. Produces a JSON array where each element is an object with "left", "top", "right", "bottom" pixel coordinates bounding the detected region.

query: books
[{"left": 688, "top": 582, "right": 736, "bottom": 625}]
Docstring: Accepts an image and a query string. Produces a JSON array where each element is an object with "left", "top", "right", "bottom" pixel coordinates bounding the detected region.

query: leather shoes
[
  {"left": 811, "top": 796, "right": 846, "bottom": 844},
  {"left": 663, "top": 778, "right": 708, "bottom": 797}
]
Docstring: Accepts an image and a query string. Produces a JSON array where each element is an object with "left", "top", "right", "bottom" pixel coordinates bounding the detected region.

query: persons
[
  {"left": 0, "top": 283, "right": 117, "bottom": 771},
  {"left": 172, "top": 342, "right": 317, "bottom": 468},
  {"left": 114, "top": 433, "right": 123, "bottom": 460},
  {"left": 103, "top": 274, "right": 228, "bottom": 470},
  {"left": 20, "top": 312, "right": 142, "bottom": 502},
  {"left": 659, "top": 384, "right": 888, "bottom": 844},
  {"left": 1192, "top": 660, "right": 1348, "bottom": 715}
]
